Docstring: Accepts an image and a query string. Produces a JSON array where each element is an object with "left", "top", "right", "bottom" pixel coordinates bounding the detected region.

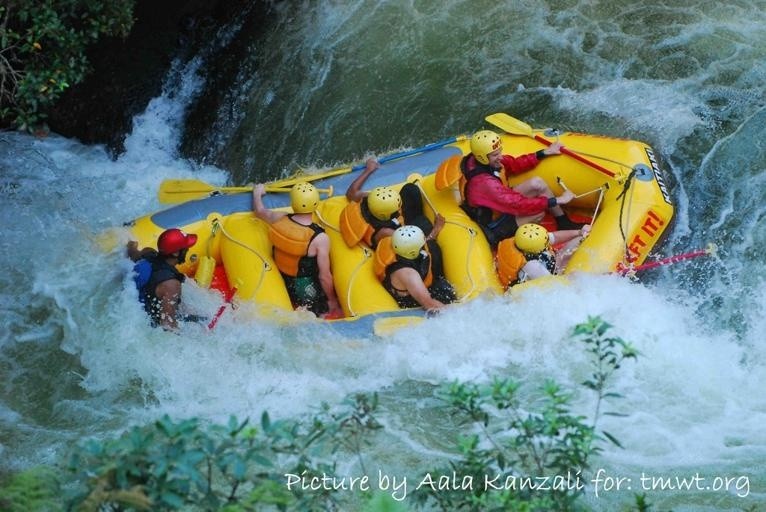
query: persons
[
  {"left": 458, "top": 130, "right": 593, "bottom": 244},
  {"left": 382, "top": 226, "right": 454, "bottom": 310},
  {"left": 345, "top": 158, "right": 444, "bottom": 249},
  {"left": 126, "top": 228, "right": 210, "bottom": 337},
  {"left": 494, "top": 223, "right": 593, "bottom": 295},
  {"left": 251, "top": 182, "right": 339, "bottom": 319}
]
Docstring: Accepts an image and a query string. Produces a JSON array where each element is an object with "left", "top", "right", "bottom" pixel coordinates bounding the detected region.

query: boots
[{"left": 553, "top": 211, "right": 590, "bottom": 232}]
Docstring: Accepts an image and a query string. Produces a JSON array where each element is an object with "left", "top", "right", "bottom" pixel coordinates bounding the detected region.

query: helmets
[
  {"left": 289, "top": 181, "right": 320, "bottom": 215},
  {"left": 514, "top": 223, "right": 550, "bottom": 255},
  {"left": 156, "top": 228, "right": 198, "bottom": 256},
  {"left": 391, "top": 224, "right": 428, "bottom": 261},
  {"left": 367, "top": 186, "right": 404, "bottom": 221},
  {"left": 469, "top": 129, "right": 503, "bottom": 166}
]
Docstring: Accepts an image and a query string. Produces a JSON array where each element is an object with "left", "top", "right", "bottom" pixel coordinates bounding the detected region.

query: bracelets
[{"left": 429, "top": 232, "right": 437, "bottom": 240}]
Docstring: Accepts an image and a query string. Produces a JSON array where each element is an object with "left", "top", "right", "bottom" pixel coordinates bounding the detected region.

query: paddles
[
  {"left": 502, "top": 246, "right": 718, "bottom": 305},
  {"left": 374, "top": 317, "right": 425, "bottom": 338},
  {"left": 273, "top": 136, "right": 466, "bottom": 187},
  {"left": 160, "top": 181, "right": 331, "bottom": 193},
  {"left": 485, "top": 112, "right": 614, "bottom": 179}
]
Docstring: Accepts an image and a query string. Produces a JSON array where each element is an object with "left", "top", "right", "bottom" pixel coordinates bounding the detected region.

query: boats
[{"left": 123, "top": 127, "right": 677, "bottom": 340}]
